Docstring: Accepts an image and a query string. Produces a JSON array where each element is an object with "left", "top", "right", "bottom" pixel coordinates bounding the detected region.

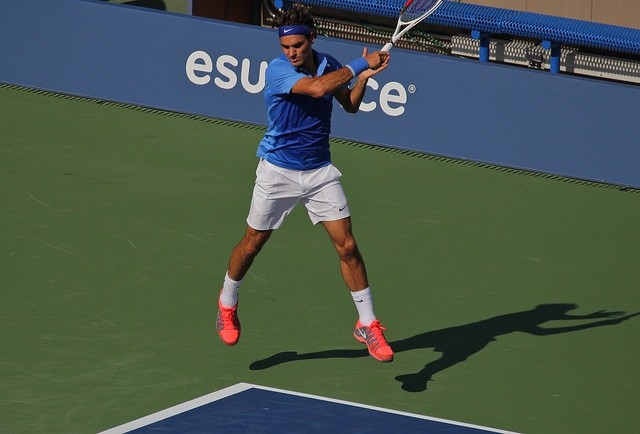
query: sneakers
[
  {"left": 353, "top": 319, "right": 394, "bottom": 363},
  {"left": 216, "top": 288, "right": 241, "bottom": 346}
]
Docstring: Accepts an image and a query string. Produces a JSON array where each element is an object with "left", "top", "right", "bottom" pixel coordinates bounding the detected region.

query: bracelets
[{"left": 344, "top": 56, "right": 370, "bottom": 78}]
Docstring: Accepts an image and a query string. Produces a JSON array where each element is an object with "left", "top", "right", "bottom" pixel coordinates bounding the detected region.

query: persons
[{"left": 215, "top": 5, "right": 394, "bottom": 363}]
